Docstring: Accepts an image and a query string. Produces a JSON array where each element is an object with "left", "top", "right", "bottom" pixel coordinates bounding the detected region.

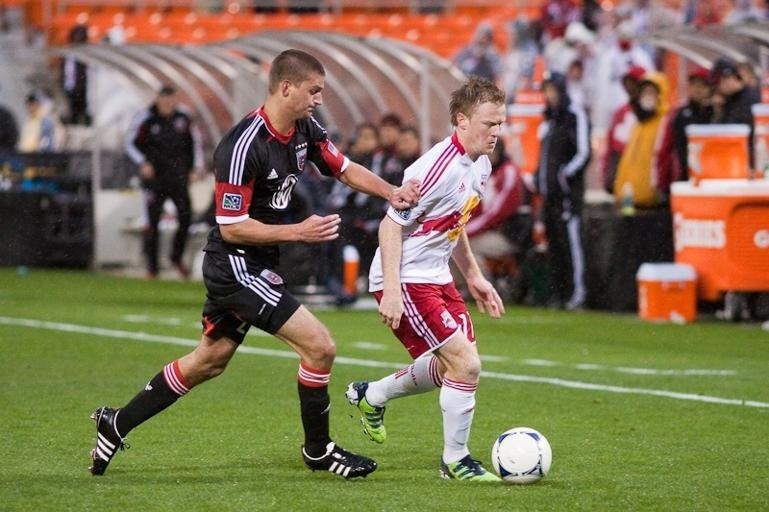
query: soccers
[{"left": 492, "top": 428, "right": 553, "bottom": 484}]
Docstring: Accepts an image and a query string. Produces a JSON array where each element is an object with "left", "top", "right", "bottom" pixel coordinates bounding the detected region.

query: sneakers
[
  {"left": 439, "top": 454, "right": 504, "bottom": 485},
  {"left": 89, "top": 407, "right": 131, "bottom": 476},
  {"left": 346, "top": 381, "right": 388, "bottom": 445},
  {"left": 300, "top": 443, "right": 377, "bottom": 480}
]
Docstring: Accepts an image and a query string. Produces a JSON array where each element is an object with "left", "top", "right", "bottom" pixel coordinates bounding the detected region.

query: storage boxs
[
  {"left": 635, "top": 260, "right": 700, "bottom": 324},
  {"left": 670, "top": 104, "right": 769, "bottom": 321}
]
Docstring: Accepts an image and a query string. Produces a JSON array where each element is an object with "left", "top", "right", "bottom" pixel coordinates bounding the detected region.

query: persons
[
  {"left": 60, "top": 23, "right": 94, "bottom": 126},
  {"left": 458, "top": 0, "right": 768, "bottom": 322},
  {"left": 278, "top": 112, "right": 421, "bottom": 307},
  {"left": 344, "top": 75, "right": 502, "bottom": 483},
  {"left": 89, "top": 49, "right": 422, "bottom": 482},
  {"left": 121, "top": 84, "right": 204, "bottom": 280},
  {"left": 14, "top": 88, "right": 68, "bottom": 154}
]
